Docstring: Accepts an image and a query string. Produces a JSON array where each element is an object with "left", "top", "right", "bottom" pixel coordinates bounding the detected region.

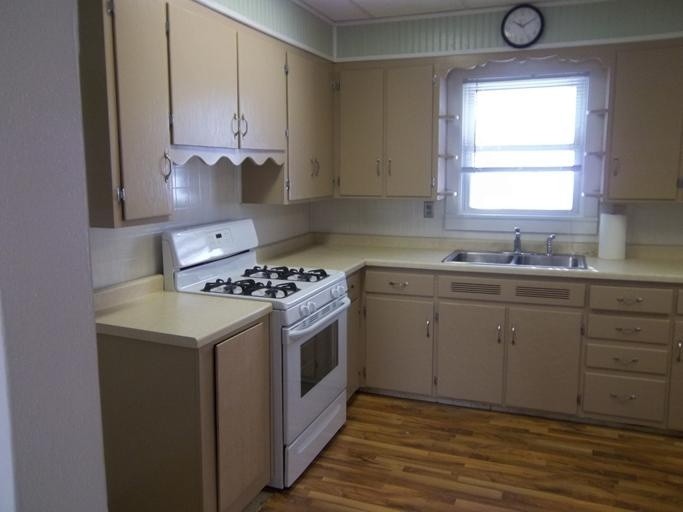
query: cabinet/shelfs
[
  {"left": 95, "top": 314, "right": 274, "bottom": 511},
  {"left": 77, "top": 0, "right": 179, "bottom": 229},
  {"left": 344, "top": 266, "right": 362, "bottom": 404},
  {"left": 333, "top": 59, "right": 447, "bottom": 202},
  {"left": 584, "top": 36, "right": 682, "bottom": 203},
  {"left": 433, "top": 269, "right": 585, "bottom": 426},
  {"left": 168, "top": 0, "right": 286, "bottom": 168},
  {"left": 242, "top": 37, "right": 331, "bottom": 207},
  {"left": 362, "top": 262, "right": 432, "bottom": 404},
  {"left": 583, "top": 274, "right": 681, "bottom": 436}
]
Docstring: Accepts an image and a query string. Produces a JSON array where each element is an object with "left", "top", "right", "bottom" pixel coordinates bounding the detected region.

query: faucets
[
  {"left": 545, "top": 234, "right": 556, "bottom": 255},
  {"left": 514, "top": 225, "right": 523, "bottom": 252}
]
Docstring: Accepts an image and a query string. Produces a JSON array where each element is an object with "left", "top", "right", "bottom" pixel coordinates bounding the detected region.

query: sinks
[
  {"left": 515, "top": 251, "right": 587, "bottom": 269},
  {"left": 442, "top": 250, "right": 514, "bottom": 266}
]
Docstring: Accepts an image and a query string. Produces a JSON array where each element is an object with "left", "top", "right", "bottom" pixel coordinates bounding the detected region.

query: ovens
[{"left": 260, "top": 296, "right": 349, "bottom": 493}]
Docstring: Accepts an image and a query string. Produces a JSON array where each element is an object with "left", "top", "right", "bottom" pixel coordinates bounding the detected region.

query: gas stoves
[{"left": 183, "top": 261, "right": 350, "bottom": 325}]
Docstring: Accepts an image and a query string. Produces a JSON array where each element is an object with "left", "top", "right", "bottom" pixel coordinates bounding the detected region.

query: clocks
[{"left": 499, "top": 4, "right": 545, "bottom": 48}]
